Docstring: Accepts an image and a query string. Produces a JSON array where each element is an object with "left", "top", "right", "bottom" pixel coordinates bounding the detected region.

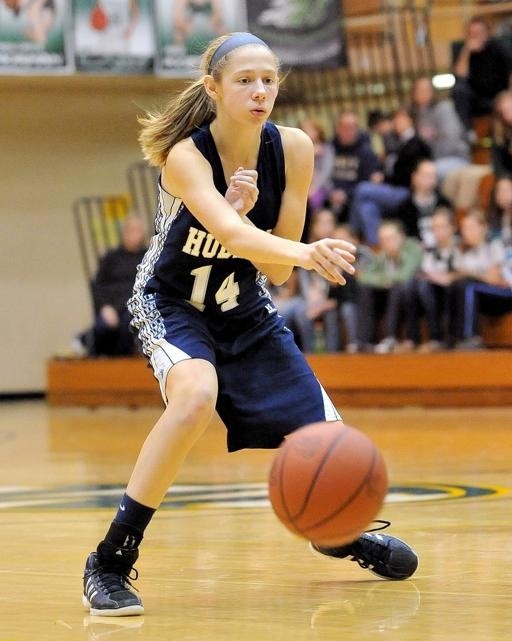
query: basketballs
[{"left": 269, "top": 421, "right": 387, "bottom": 548}]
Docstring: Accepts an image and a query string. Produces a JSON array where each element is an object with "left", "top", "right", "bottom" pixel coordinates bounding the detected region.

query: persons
[
  {"left": 170, "top": 0, "right": 229, "bottom": 43},
  {"left": 91, "top": 215, "right": 155, "bottom": 356},
  {"left": 92, "top": 0, "right": 140, "bottom": 38},
  {"left": 80, "top": 29, "right": 420, "bottom": 618},
  {"left": 27, "top": 0, "right": 58, "bottom": 44}
]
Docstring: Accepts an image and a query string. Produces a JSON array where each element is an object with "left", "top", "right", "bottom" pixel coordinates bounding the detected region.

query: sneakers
[
  {"left": 308, "top": 530, "right": 419, "bottom": 581},
  {"left": 82, "top": 539, "right": 144, "bottom": 617}
]
